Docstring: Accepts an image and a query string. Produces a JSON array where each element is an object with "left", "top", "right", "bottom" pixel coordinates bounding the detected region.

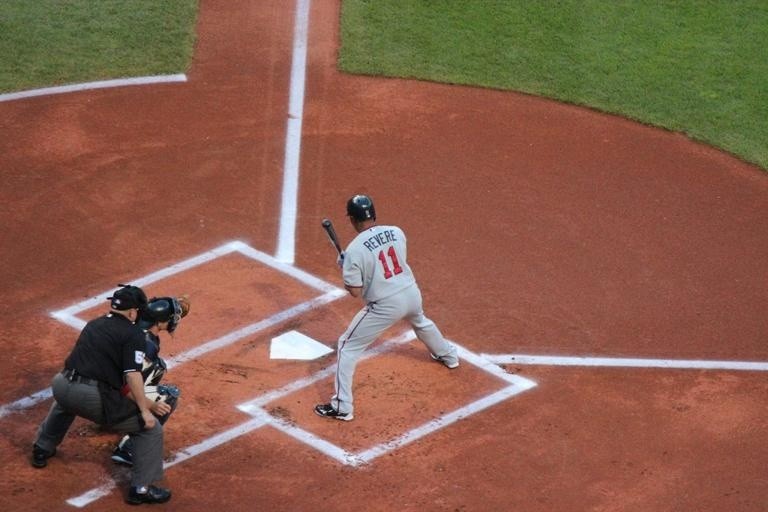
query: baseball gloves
[{"left": 177, "top": 295, "right": 191, "bottom": 318}]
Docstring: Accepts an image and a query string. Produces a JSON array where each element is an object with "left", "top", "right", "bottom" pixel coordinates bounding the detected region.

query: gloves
[{"left": 335, "top": 250, "right": 348, "bottom": 270}]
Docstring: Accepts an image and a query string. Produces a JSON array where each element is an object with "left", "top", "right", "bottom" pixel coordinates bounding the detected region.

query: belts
[{"left": 60, "top": 366, "right": 98, "bottom": 390}]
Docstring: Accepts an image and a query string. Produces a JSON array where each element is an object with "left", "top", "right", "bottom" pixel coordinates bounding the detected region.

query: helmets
[
  {"left": 345, "top": 193, "right": 376, "bottom": 222},
  {"left": 136, "top": 296, "right": 177, "bottom": 331}
]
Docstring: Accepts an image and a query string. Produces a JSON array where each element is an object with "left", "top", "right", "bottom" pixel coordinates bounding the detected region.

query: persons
[
  {"left": 311, "top": 192, "right": 460, "bottom": 424},
  {"left": 28, "top": 282, "right": 176, "bottom": 506},
  {"left": 107, "top": 292, "right": 197, "bottom": 468}
]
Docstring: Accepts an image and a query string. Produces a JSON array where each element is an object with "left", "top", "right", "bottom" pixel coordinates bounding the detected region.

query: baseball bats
[{"left": 322, "top": 218, "right": 345, "bottom": 260}]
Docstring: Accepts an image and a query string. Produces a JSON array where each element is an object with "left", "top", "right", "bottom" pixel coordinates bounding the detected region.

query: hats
[{"left": 110, "top": 285, "right": 146, "bottom": 312}]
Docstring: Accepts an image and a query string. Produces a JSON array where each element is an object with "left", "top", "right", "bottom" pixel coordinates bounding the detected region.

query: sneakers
[
  {"left": 429, "top": 350, "right": 460, "bottom": 371},
  {"left": 30, "top": 445, "right": 52, "bottom": 467},
  {"left": 126, "top": 483, "right": 171, "bottom": 506},
  {"left": 313, "top": 402, "right": 355, "bottom": 423},
  {"left": 110, "top": 446, "right": 137, "bottom": 467}
]
